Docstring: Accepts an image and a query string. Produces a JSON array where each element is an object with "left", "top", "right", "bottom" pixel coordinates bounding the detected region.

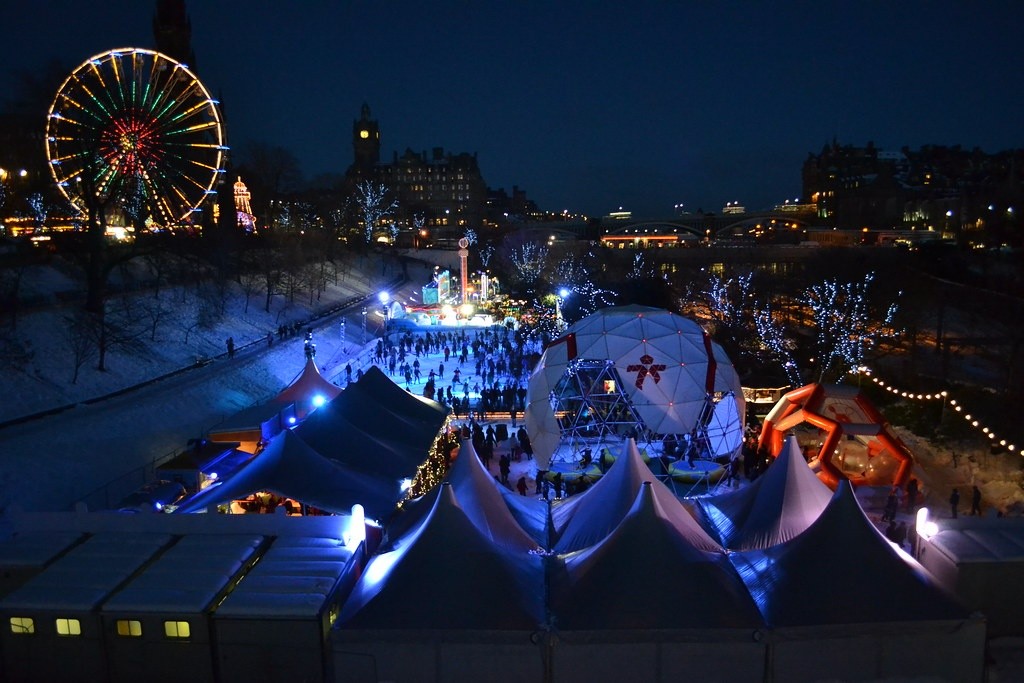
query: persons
[
  {"left": 969, "top": 486, "right": 981, "bottom": 516},
  {"left": 227, "top": 337, "right": 234, "bottom": 358},
  {"left": 802, "top": 446, "right": 809, "bottom": 461},
  {"left": 267, "top": 331, "right": 273, "bottom": 347},
  {"left": 907, "top": 479, "right": 918, "bottom": 511},
  {"left": 886, "top": 521, "right": 906, "bottom": 548},
  {"left": 344, "top": 326, "right": 767, "bottom": 499},
  {"left": 881, "top": 494, "right": 898, "bottom": 522},
  {"left": 949, "top": 489, "right": 960, "bottom": 519},
  {"left": 278, "top": 321, "right": 302, "bottom": 341}
]
